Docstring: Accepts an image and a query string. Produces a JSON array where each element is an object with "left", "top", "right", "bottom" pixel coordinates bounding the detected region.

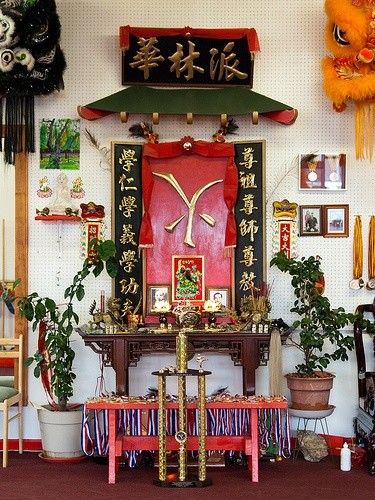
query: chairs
[{"left": 0, "top": 334, "right": 23, "bottom": 467}]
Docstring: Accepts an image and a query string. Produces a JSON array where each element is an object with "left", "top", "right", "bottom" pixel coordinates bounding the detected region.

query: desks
[
  {"left": 86, "top": 399, "right": 289, "bottom": 484},
  {"left": 79, "top": 327, "right": 287, "bottom": 395}
]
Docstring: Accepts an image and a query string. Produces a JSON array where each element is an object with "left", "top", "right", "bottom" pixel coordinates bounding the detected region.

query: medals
[
  {"left": 86, "top": 393, "right": 283, "bottom": 404},
  {"left": 329, "top": 171, "right": 338, "bottom": 181},
  {"left": 366, "top": 278, "right": 375, "bottom": 291},
  {"left": 349, "top": 278, "right": 364, "bottom": 290},
  {"left": 308, "top": 172, "right": 317, "bottom": 181}
]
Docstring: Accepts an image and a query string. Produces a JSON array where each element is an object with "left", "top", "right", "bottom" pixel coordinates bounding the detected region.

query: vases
[
  {"left": 208, "top": 312, "right": 217, "bottom": 323},
  {"left": 159, "top": 314, "right": 168, "bottom": 324}
]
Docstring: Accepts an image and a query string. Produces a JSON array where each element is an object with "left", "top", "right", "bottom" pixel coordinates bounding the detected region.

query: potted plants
[
  {"left": 0, "top": 237, "right": 118, "bottom": 458},
  {"left": 271, "top": 251, "right": 375, "bottom": 409}
]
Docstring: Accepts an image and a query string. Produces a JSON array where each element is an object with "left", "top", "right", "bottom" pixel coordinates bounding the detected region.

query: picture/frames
[
  {"left": 146, "top": 284, "right": 173, "bottom": 314},
  {"left": 206, "top": 287, "right": 231, "bottom": 316},
  {"left": 300, "top": 204, "right": 349, "bottom": 237}
]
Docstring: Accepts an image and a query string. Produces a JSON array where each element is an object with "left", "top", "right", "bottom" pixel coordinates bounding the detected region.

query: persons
[
  {"left": 152, "top": 290, "right": 165, "bottom": 309},
  {"left": 305, "top": 212, "right": 319, "bottom": 232},
  {"left": 304, "top": 210, "right": 311, "bottom": 227},
  {"left": 213, "top": 292, "right": 225, "bottom": 310}
]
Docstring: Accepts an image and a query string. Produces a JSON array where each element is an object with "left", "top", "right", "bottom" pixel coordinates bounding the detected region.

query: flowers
[
  {"left": 202, "top": 300, "right": 223, "bottom": 314},
  {"left": 151, "top": 300, "right": 172, "bottom": 313}
]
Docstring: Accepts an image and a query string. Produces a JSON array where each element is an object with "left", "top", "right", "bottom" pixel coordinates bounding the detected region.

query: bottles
[{"left": 340, "top": 442, "right": 351, "bottom": 471}]
